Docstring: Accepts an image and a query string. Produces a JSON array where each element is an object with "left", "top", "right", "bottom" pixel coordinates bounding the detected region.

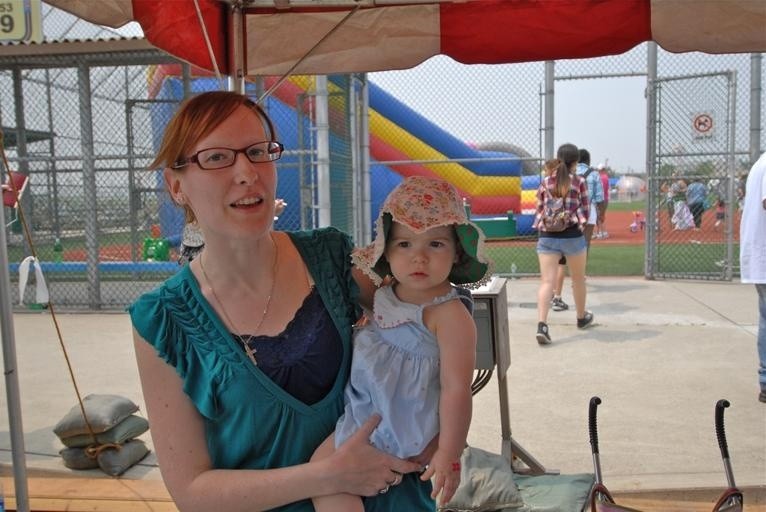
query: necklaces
[{"left": 190, "top": 236, "right": 287, "bottom": 368}]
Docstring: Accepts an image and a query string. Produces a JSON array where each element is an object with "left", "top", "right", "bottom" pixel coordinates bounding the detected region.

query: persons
[
  {"left": 533, "top": 143, "right": 594, "bottom": 348},
  {"left": 304, "top": 175, "right": 479, "bottom": 509},
  {"left": 658, "top": 168, "right": 747, "bottom": 232},
  {"left": 548, "top": 147, "right": 609, "bottom": 310},
  {"left": 738, "top": 147, "right": 766, "bottom": 401},
  {"left": 595, "top": 161, "right": 609, "bottom": 241},
  {"left": 126, "top": 89, "right": 427, "bottom": 512},
  {"left": 273, "top": 193, "right": 288, "bottom": 220}
]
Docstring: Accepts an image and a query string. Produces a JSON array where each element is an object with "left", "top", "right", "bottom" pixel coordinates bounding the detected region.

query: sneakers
[
  {"left": 536, "top": 322, "right": 551, "bottom": 343},
  {"left": 552, "top": 298, "right": 567, "bottom": 310},
  {"left": 577, "top": 312, "right": 592, "bottom": 327}
]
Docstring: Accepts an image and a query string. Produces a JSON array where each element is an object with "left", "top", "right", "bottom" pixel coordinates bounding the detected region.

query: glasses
[{"left": 175, "top": 140, "right": 284, "bottom": 170}]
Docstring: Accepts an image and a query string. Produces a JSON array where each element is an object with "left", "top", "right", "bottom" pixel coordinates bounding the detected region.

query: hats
[{"left": 350, "top": 177, "right": 494, "bottom": 289}]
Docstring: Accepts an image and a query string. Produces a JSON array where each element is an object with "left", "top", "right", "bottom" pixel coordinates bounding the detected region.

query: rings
[
  {"left": 387, "top": 472, "right": 406, "bottom": 488},
  {"left": 375, "top": 483, "right": 392, "bottom": 497}
]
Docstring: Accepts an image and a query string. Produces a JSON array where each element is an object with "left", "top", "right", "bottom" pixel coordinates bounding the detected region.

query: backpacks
[{"left": 538, "top": 192, "right": 573, "bottom": 232}]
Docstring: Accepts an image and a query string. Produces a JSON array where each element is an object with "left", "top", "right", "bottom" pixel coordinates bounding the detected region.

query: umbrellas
[{"left": 35, "top": 1, "right": 766, "bottom": 102}]
